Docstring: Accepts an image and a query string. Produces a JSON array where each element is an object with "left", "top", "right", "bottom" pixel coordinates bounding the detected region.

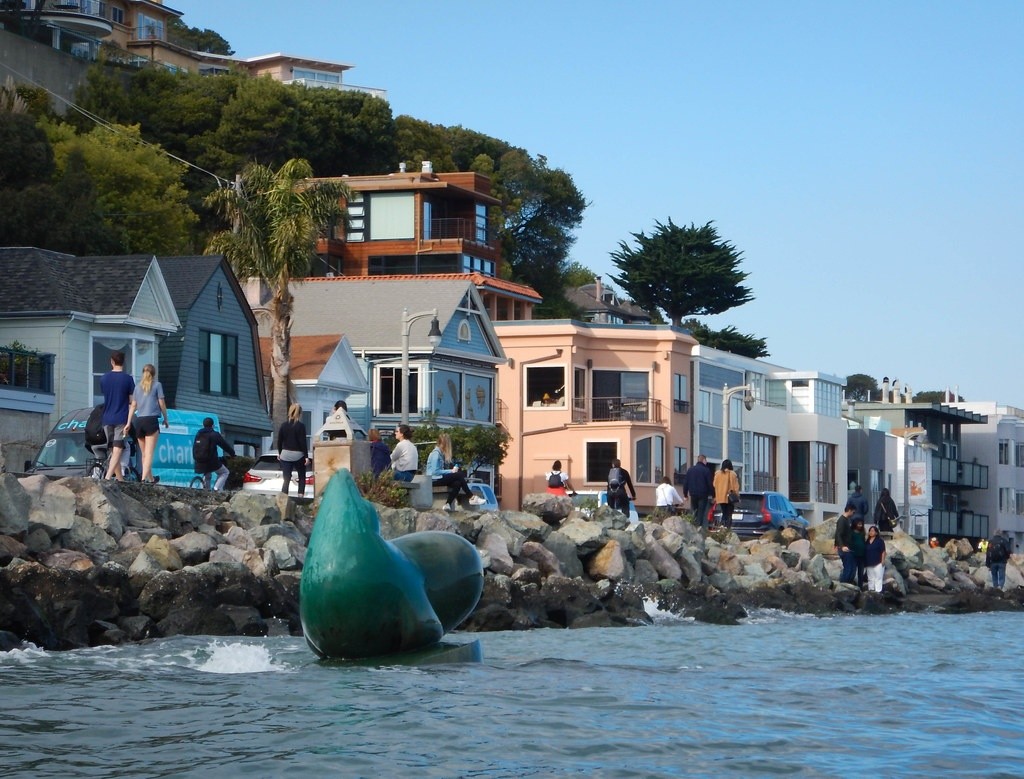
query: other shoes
[
  {"left": 468, "top": 496, "right": 487, "bottom": 505},
  {"left": 443, "top": 503, "right": 451, "bottom": 510},
  {"left": 141, "top": 476, "right": 160, "bottom": 486}
]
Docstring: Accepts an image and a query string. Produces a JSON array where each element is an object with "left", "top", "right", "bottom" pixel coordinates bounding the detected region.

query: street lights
[
  {"left": 722, "top": 382, "right": 755, "bottom": 469},
  {"left": 400, "top": 307, "right": 442, "bottom": 425}
]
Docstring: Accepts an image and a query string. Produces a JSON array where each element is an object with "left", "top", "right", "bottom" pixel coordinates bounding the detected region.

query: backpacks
[
  {"left": 548, "top": 472, "right": 563, "bottom": 488},
  {"left": 608, "top": 467, "right": 627, "bottom": 492},
  {"left": 193, "top": 430, "right": 216, "bottom": 462},
  {"left": 85, "top": 405, "right": 108, "bottom": 446},
  {"left": 987, "top": 538, "right": 1010, "bottom": 563}
]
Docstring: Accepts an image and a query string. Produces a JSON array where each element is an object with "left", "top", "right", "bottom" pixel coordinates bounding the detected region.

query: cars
[
  {"left": 243, "top": 449, "right": 315, "bottom": 504},
  {"left": 567, "top": 489, "right": 638, "bottom": 526}
]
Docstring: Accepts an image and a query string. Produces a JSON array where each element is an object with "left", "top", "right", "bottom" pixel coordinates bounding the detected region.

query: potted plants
[{"left": 0, "top": 339, "right": 40, "bottom": 387}]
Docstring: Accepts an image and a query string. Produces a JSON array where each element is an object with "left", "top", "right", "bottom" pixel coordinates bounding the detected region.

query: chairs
[{"left": 607, "top": 399, "right": 623, "bottom": 421}]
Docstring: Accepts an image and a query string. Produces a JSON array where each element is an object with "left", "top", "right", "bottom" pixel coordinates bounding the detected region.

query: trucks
[{"left": 23, "top": 405, "right": 227, "bottom": 491}]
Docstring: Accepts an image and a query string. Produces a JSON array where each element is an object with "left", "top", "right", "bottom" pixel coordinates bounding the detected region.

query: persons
[
  {"left": 329, "top": 400, "right": 348, "bottom": 416},
  {"left": 390, "top": 424, "right": 418, "bottom": 482},
  {"left": 847, "top": 486, "right": 869, "bottom": 518},
  {"left": 546, "top": 461, "right": 577, "bottom": 497},
  {"left": 277, "top": 404, "right": 309, "bottom": 497},
  {"left": 368, "top": 429, "right": 392, "bottom": 477},
  {"left": 100, "top": 352, "right": 136, "bottom": 480},
  {"left": 194, "top": 418, "right": 235, "bottom": 491},
  {"left": 835, "top": 504, "right": 886, "bottom": 592},
  {"left": 874, "top": 489, "right": 899, "bottom": 532},
  {"left": 656, "top": 476, "right": 684, "bottom": 515},
  {"left": 985, "top": 528, "right": 1006, "bottom": 590},
  {"left": 713, "top": 459, "right": 739, "bottom": 528},
  {"left": 607, "top": 459, "right": 636, "bottom": 518},
  {"left": 123, "top": 364, "right": 170, "bottom": 484},
  {"left": 978, "top": 539, "right": 988, "bottom": 552},
  {"left": 683, "top": 455, "right": 715, "bottom": 524},
  {"left": 427, "top": 434, "right": 486, "bottom": 510}
]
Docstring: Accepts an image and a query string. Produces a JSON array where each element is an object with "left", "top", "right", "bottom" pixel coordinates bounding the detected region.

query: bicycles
[
  {"left": 189, "top": 456, "right": 232, "bottom": 492},
  {"left": 656, "top": 503, "right": 684, "bottom": 524},
  {"left": 85, "top": 444, "right": 142, "bottom": 482},
  {"left": 610, "top": 497, "right": 637, "bottom": 515}
]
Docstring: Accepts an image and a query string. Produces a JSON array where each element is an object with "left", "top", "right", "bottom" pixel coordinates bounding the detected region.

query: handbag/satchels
[
  {"left": 728, "top": 494, "right": 740, "bottom": 504},
  {"left": 887, "top": 518, "right": 897, "bottom": 529},
  {"left": 666, "top": 504, "right": 681, "bottom": 517}
]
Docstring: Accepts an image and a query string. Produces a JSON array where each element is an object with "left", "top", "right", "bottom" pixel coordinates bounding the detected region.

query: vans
[{"left": 465, "top": 477, "right": 499, "bottom": 512}]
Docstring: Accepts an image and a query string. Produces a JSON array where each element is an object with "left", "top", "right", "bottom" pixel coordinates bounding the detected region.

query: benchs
[{"left": 373, "top": 475, "right": 468, "bottom": 510}]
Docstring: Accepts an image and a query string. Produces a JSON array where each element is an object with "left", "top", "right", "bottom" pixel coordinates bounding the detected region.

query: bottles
[{"left": 453, "top": 463, "right": 458, "bottom": 472}]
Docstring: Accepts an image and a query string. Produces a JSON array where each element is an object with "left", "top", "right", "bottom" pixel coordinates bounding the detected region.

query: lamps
[
  {"left": 662, "top": 349, "right": 668, "bottom": 360},
  {"left": 508, "top": 358, "right": 512, "bottom": 367},
  {"left": 587, "top": 359, "right": 592, "bottom": 369},
  {"left": 651, "top": 361, "right": 656, "bottom": 370}
]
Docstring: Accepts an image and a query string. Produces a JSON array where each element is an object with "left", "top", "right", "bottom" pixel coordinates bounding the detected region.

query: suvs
[{"left": 707, "top": 490, "right": 810, "bottom": 542}]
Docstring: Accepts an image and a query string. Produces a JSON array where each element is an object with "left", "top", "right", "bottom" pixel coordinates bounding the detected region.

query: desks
[{"left": 623, "top": 403, "right": 641, "bottom": 420}]
